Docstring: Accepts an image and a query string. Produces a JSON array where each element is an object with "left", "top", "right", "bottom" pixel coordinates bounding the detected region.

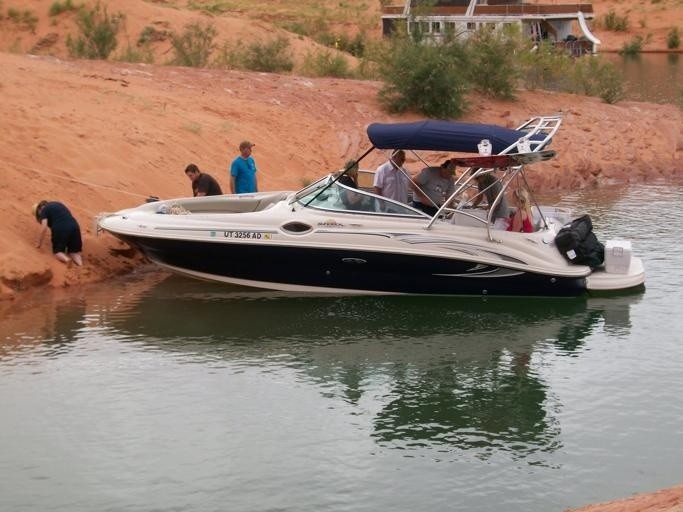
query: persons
[
  {"left": 229, "top": 140, "right": 259, "bottom": 194},
  {"left": 337, "top": 158, "right": 375, "bottom": 211},
  {"left": 505, "top": 187, "right": 534, "bottom": 233},
  {"left": 41, "top": 287, "right": 89, "bottom": 361},
  {"left": 409, "top": 159, "right": 455, "bottom": 220},
  {"left": 372, "top": 148, "right": 429, "bottom": 216},
  {"left": 30, "top": 198, "right": 83, "bottom": 267},
  {"left": 469, "top": 166, "right": 510, "bottom": 223},
  {"left": 184, "top": 163, "right": 223, "bottom": 197}
]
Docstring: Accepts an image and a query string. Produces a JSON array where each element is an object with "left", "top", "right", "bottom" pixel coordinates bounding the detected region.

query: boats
[{"left": 95, "top": 107, "right": 647, "bottom": 297}]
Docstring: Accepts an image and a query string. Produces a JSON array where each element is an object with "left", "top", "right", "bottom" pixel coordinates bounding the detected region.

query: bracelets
[{"left": 472, "top": 203, "right": 476, "bottom": 207}]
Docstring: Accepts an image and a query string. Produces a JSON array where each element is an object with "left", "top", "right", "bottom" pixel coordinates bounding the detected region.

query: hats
[
  {"left": 32, "top": 200, "right": 47, "bottom": 221},
  {"left": 441, "top": 160, "right": 456, "bottom": 175},
  {"left": 239, "top": 141, "right": 255, "bottom": 151}
]
